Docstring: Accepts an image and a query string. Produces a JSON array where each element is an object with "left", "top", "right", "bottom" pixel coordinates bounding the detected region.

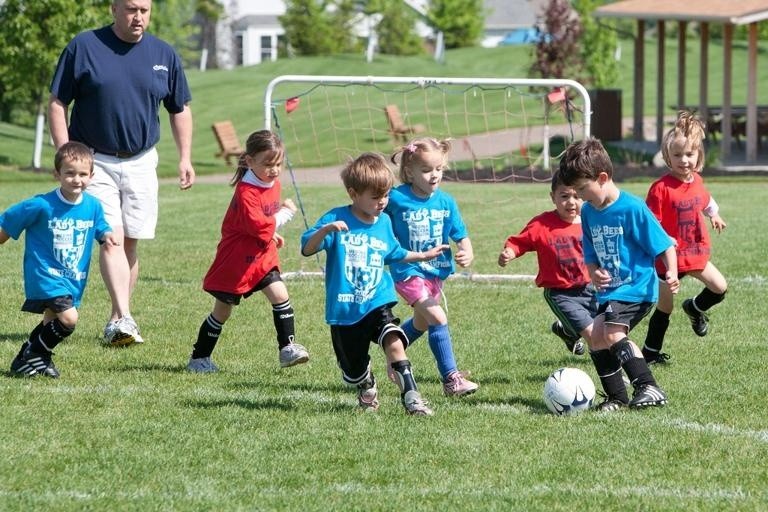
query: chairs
[
  {"left": 210, "top": 120, "right": 245, "bottom": 166},
  {"left": 667, "top": 104, "right": 768, "bottom": 152},
  {"left": 382, "top": 104, "right": 423, "bottom": 140}
]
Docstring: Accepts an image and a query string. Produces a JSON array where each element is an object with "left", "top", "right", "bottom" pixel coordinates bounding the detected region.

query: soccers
[{"left": 544, "top": 368, "right": 595, "bottom": 416}]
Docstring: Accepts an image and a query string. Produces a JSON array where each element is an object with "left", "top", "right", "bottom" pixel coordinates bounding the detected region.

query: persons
[
  {"left": 49, "top": 0, "right": 203, "bottom": 348},
  {"left": 381, "top": 137, "right": 479, "bottom": 402},
  {"left": 0, "top": 142, "right": 122, "bottom": 380},
  {"left": 559, "top": 136, "right": 681, "bottom": 412},
  {"left": 639, "top": 114, "right": 730, "bottom": 363},
  {"left": 300, "top": 151, "right": 435, "bottom": 418},
  {"left": 496, "top": 168, "right": 601, "bottom": 355},
  {"left": 185, "top": 129, "right": 312, "bottom": 371}
]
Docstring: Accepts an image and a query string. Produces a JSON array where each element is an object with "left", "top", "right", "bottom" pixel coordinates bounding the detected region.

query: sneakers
[
  {"left": 628, "top": 383, "right": 668, "bottom": 410},
  {"left": 552, "top": 319, "right": 585, "bottom": 355},
  {"left": 354, "top": 372, "right": 378, "bottom": 412},
  {"left": 10, "top": 351, "right": 39, "bottom": 377},
  {"left": 385, "top": 356, "right": 396, "bottom": 384},
  {"left": 102, "top": 315, "right": 145, "bottom": 347},
  {"left": 683, "top": 297, "right": 710, "bottom": 337},
  {"left": 640, "top": 348, "right": 671, "bottom": 367},
  {"left": 279, "top": 334, "right": 310, "bottom": 369},
  {"left": 400, "top": 390, "right": 433, "bottom": 417},
  {"left": 23, "top": 347, "right": 59, "bottom": 379},
  {"left": 593, "top": 390, "right": 631, "bottom": 413},
  {"left": 443, "top": 370, "right": 479, "bottom": 399},
  {"left": 187, "top": 354, "right": 221, "bottom": 373}
]
